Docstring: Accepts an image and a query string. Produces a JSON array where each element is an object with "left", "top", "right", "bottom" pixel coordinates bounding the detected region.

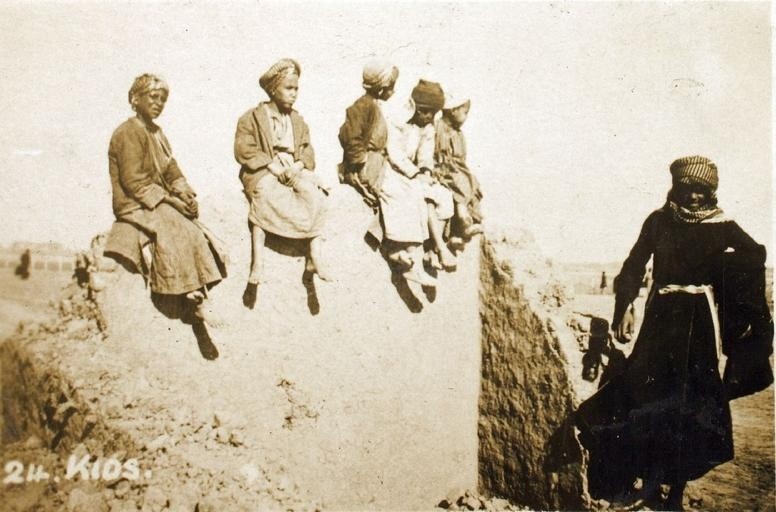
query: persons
[
  {"left": 337, "top": 58, "right": 482, "bottom": 287},
  {"left": 234, "top": 56, "right": 339, "bottom": 284},
  {"left": 575, "top": 157, "right": 776, "bottom": 512},
  {"left": 104, "top": 72, "right": 233, "bottom": 328}
]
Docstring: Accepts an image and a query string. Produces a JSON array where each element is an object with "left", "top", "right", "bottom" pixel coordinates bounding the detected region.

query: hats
[
  {"left": 258, "top": 57, "right": 301, "bottom": 95},
  {"left": 412, "top": 80, "right": 446, "bottom": 111},
  {"left": 361, "top": 58, "right": 395, "bottom": 91},
  {"left": 670, "top": 156, "right": 718, "bottom": 193},
  {"left": 126, "top": 72, "right": 169, "bottom": 103},
  {"left": 441, "top": 79, "right": 471, "bottom": 109}
]
